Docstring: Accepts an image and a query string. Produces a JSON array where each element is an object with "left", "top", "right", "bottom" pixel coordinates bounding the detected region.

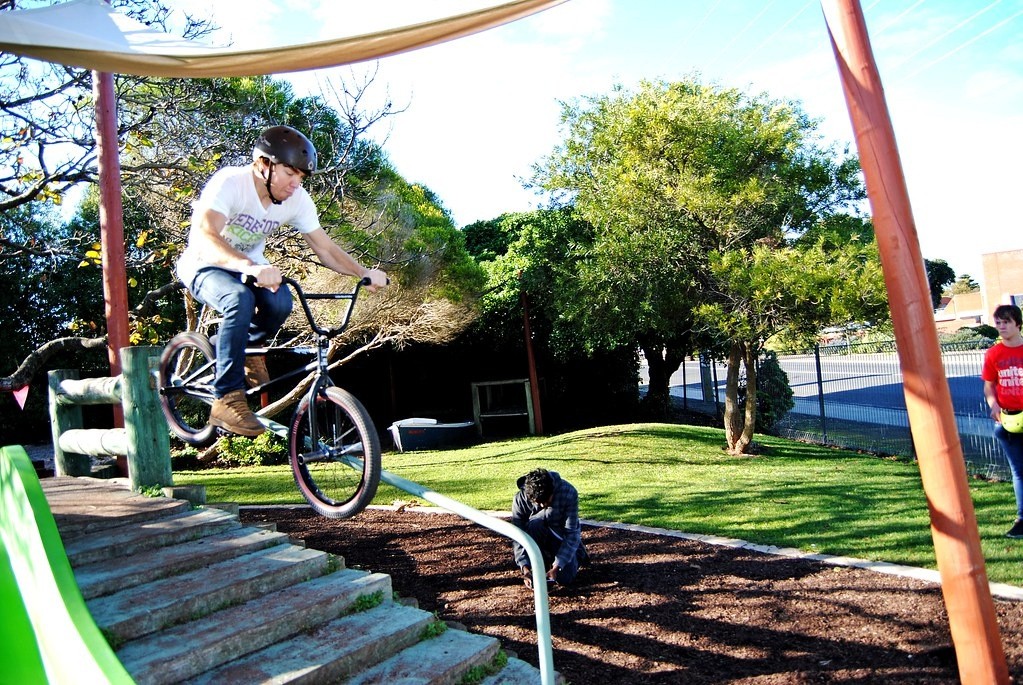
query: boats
[{"left": 391, "top": 409, "right": 480, "bottom": 451}]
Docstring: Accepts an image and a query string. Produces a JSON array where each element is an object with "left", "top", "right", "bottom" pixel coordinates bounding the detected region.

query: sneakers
[
  {"left": 209, "top": 391, "right": 264, "bottom": 436},
  {"left": 244, "top": 355, "right": 270, "bottom": 388},
  {"left": 1006, "top": 517, "right": 1023, "bottom": 538}
]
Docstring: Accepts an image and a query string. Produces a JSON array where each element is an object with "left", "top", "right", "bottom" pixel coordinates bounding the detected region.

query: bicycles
[{"left": 156, "top": 275, "right": 390, "bottom": 519}]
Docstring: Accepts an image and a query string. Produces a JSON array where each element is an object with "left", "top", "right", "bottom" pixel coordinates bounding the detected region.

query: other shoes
[
  {"left": 547, "top": 578, "right": 556, "bottom": 594},
  {"left": 576, "top": 540, "right": 591, "bottom": 568}
]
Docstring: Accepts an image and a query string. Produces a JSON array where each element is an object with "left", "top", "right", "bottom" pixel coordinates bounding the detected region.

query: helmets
[{"left": 252, "top": 125, "right": 317, "bottom": 176}]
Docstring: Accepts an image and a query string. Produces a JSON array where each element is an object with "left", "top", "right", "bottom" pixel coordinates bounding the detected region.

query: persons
[
  {"left": 981, "top": 305, "right": 1023, "bottom": 538},
  {"left": 175, "top": 126, "right": 387, "bottom": 437},
  {"left": 511, "top": 468, "right": 590, "bottom": 589}
]
